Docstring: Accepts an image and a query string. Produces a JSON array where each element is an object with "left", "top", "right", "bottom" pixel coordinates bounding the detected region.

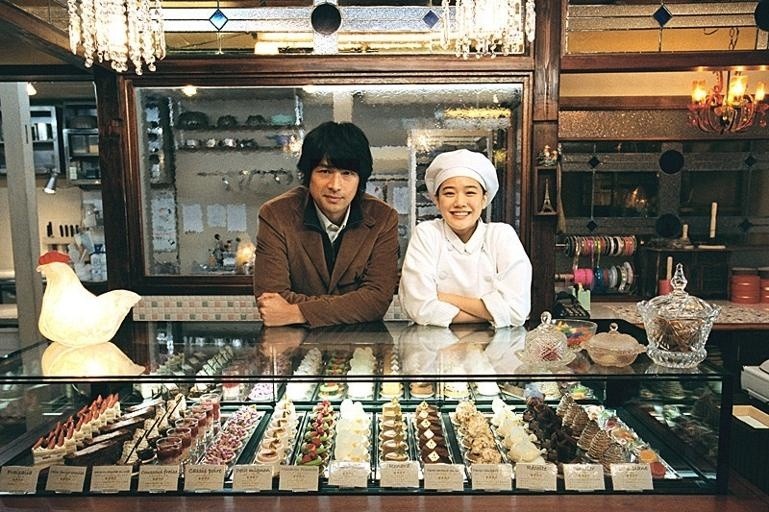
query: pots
[{"left": 178, "top": 136, "right": 261, "bottom": 149}]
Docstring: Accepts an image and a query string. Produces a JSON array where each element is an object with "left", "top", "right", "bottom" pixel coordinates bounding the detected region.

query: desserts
[
  {"left": 30, "top": 394, "right": 666, "bottom": 479},
  {"left": 133, "top": 342, "right": 591, "bottom": 401}
]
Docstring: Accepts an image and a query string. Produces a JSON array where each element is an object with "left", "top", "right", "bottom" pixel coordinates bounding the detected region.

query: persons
[
  {"left": 254, "top": 120, "right": 402, "bottom": 327},
  {"left": 398, "top": 147, "right": 532, "bottom": 329}
]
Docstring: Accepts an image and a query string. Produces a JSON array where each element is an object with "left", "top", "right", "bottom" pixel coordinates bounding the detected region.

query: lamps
[
  {"left": 686, "top": 66, "right": 769, "bottom": 136},
  {"left": 42, "top": 170, "right": 65, "bottom": 195}
]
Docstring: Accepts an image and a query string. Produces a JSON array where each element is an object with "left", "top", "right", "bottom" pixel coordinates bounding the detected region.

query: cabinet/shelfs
[
  {"left": 172, "top": 120, "right": 302, "bottom": 151},
  {"left": 30, "top": 106, "right": 61, "bottom": 176},
  {"left": 62, "top": 101, "right": 101, "bottom": 184},
  {"left": 134, "top": 86, "right": 304, "bottom": 277},
  {"left": 408, "top": 128, "right": 492, "bottom": 241}
]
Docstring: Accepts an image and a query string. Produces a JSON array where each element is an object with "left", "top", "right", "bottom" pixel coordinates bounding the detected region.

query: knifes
[{"left": 45, "top": 221, "right": 83, "bottom": 255}]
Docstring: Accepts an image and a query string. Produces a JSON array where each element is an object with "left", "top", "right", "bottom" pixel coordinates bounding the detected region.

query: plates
[{"left": 513, "top": 346, "right": 578, "bottom": 369}]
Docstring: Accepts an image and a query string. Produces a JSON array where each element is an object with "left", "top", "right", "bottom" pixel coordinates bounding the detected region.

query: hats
[{"left": 424, "top": 149, "right": 499, "bottom": 211}]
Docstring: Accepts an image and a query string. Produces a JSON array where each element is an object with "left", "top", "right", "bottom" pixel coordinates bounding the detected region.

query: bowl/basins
[
  {"left": 636, "top": 264, "right": 722, "bottom": 371},
  {"left": 542, "top": 317, "right": 600, "bottom": 351},
  {"left": 581, "top": 324, "right": 648, "bottom": 368}
]
